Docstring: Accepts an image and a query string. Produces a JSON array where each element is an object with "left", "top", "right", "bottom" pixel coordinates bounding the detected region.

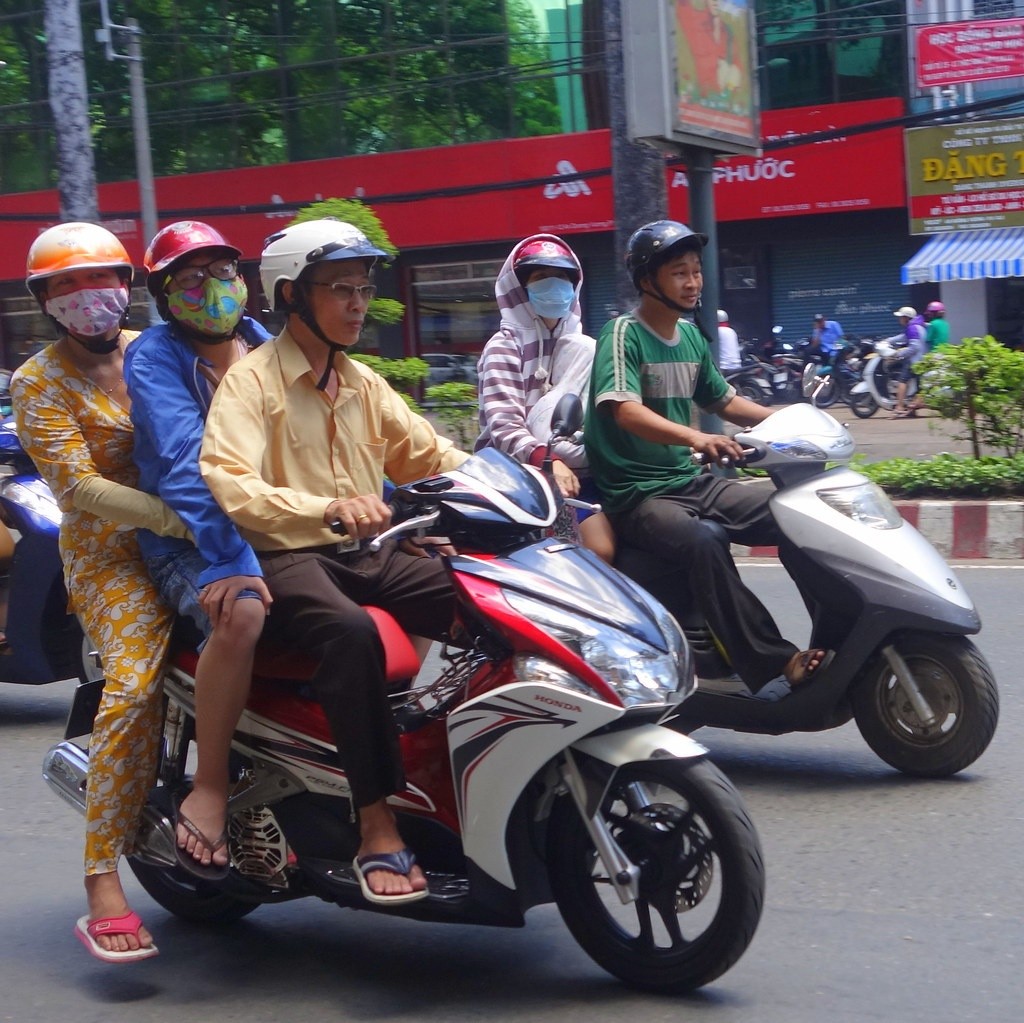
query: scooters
[
  {"left": 41, "top": 393, "right": 770, "bottom": 998},
  {"left": 562, "top": 376, "right": 1000, "bottom": 778},
  {"left": 1, "top": 367, "right": 107, "bottom": 686},
  {"left": 720, "top": 324, "right": 960, "bottom": 420}
]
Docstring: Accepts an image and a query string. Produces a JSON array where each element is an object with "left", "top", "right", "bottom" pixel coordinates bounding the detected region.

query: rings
[{"left": 355, "top": 514, "right": 367, "bottom": 523}]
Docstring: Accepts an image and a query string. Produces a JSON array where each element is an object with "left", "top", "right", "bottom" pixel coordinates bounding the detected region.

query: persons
[
  {"left": 10, "top": 222, "right": 202, "bottom": 963},
  {"left": 198, "top": 216, "right": 475, "bottom": 908},
  {"left": 475, "top": 232, "right": 614, "bottom": 567},
  {"left": 123, "top": 220, "right": 457, "bottom": 880},
  {"left": 889, "top": 302, "right": 951, "bottom": 421},
  {"left": 584, "top": 219, "right": 836, "bottom": 699},
  {"left": 716, "top": 309, "right": 741, "bottom": 378},
  {"left": 804, "top": 313, "right": 847, "bottom": 368}
]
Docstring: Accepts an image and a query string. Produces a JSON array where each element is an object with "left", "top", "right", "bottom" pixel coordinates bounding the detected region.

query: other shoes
[
  {"left": 908, "top": 401, "right": 923, "bottom": 408},
  {"left": 0, "top": 631, "right": 8, "bottom": 645},
  {"left": 890, "top": 408, "right": 908, "bottom": 420}
]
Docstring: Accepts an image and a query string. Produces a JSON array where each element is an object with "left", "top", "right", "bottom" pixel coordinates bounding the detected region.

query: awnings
[{"left": 900, "top": 226, "right": 1024, "bottom": 285}]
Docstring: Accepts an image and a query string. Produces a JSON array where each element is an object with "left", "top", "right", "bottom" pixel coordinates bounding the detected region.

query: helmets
[
  {"left": 260, "top": 218, "right": 388, "bottom": 313},
  {"left": 512, "top": 239, "right": 580, "bottom": 283},
  {"left": 25, "top": 222, "right": 134, "bottom": 311},
  {"left": 143, "top": 219, "right": 243, "bottom": 296},
  {"left": 924, "top": 301, "right": 944, "bottom": 315},
  {"left": 716, "top": 309, "right": 728, "bottom": 323},
  {"left": 625, "top": 219, "right": 710, "bottom": 292}
]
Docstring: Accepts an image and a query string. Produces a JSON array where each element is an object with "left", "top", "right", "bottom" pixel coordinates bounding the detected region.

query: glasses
[
  {"left": 298, "top": 280, "right": 378, "bottom": 302},
  {"left": 160, "top": 256, "right": 240, "bottom": 290}
]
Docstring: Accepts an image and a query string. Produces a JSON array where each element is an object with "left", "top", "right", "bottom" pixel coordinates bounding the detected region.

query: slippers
[
  {"left": 789, "top": 648, "right": 835, "bottom": 691},
  {"left": 74, "top": 911, "right": 159, "bottom": 963},
  {"left": 353, "top": 849, "right": 429, "bottom": 905},
  {"left": 172, "top": 789, "right": 230, "bottom": 881}
]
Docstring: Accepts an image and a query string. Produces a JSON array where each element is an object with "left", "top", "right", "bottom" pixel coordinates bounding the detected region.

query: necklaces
[{"left": 54, "top": 332, "right": 130, "bottom": 394}]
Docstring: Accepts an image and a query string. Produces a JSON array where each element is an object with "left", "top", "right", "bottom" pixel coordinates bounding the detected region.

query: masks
[
  {"left": 44, "top": 283, "right": 129, "bottom": 335},
  {"left": 523, "top": 277, "right": 575, "bottom": 319},
  {"left": 164, "top": 274, "right": 248, "bottom": 334}
]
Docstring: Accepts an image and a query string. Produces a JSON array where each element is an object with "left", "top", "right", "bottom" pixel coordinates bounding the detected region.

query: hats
[
  {"left": 814, "top": 314, "right": 823, "bottom": 321},
  {"left": 893, "top": 306, "right": 917, "bottom": 318}
]
